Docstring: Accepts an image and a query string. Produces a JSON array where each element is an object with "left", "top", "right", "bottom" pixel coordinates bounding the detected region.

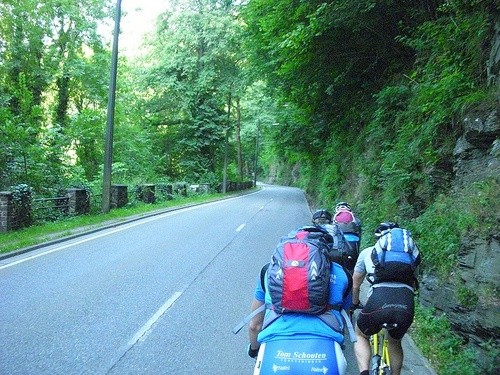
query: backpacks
[
  {"left": 333, "top": 210, "right": 360, "bottom": 237},
  {"left": 262, "top": 230, "right": 344, "bottom": 332},
  {"left": 366, "top": 228, "right": 420, "bottom": 285}
]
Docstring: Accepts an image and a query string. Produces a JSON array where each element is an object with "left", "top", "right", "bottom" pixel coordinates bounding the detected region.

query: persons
[
  {"left": 349, "top": 222, "right": 414, "bottom": 374},
  {"left": 332, "top": 203, "right": 362, "bottom": 253},
  {"left": 246, "top": 226, "right": 353, "bottom": 375},
  {"left": 311, "top": 209, "right": 352, "bottom": 268}
]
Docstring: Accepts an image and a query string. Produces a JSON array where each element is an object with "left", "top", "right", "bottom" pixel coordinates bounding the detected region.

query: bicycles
[{"left": 349, "top": 303, "right": 398, "bottom": 375}]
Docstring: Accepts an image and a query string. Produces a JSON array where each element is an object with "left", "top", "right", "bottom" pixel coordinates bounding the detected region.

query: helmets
[
  {"left": 337, "top": 202, "right": 350, "bottom": 208},
  {"left": 313, "top": 209, "right": 331, "bottom": 220},
  {"left": 375, "top": 221, "right": 399, "bottom": 233}
]
